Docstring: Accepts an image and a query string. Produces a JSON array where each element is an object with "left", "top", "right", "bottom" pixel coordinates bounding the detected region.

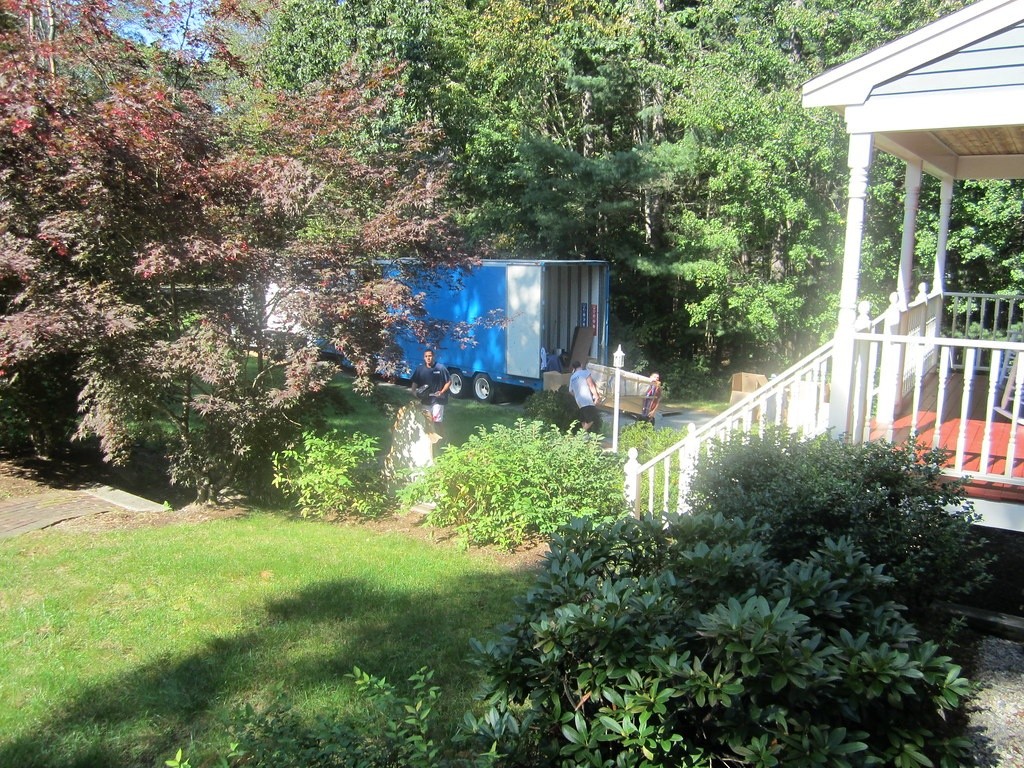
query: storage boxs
[
  {"left": 729, "top": 391, "right": 751, "bottom": 413},
  {"left": 755, "top": 375, "right": 768, "bottom": 386},
  {"left": 728, "top": 372, "right": 758, "bottom": 392},
  {"left": 543, "top": 370, "right": 572, "bottom": 392}
]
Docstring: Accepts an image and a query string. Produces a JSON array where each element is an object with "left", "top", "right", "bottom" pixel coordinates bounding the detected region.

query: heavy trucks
[{"left": 228, "top": 256, "right": 613, "bottom": 403}]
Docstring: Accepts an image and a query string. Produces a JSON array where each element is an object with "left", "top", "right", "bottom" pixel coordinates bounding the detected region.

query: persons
[
  {"left": 410, "top": 350, "right": 452, "bottom": 439},
  {"left": 636, "top": 371, "right": 664, "bottom": 430},
  {"left": 546, "top": 351, "right": 571, "bottom": 374},
  {"left": 569, "top": 360, "right": 600, "bottom": 431}
]
